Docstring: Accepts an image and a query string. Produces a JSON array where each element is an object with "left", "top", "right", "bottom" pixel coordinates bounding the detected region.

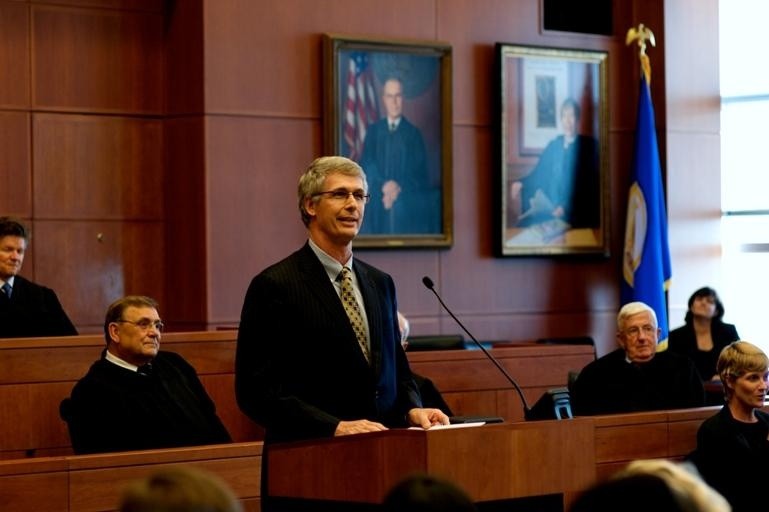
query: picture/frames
[
  {"left": 494, "top": 41, "right": 612, "bottom": 260},
  {"left": 319, "top": 32, "right": 453, "bottom": 250}
]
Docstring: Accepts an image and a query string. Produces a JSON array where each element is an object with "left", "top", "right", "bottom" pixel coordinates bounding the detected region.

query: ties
[
  {"left": 340, "top": 268, "right": 370, "bottom": 364},
  {"left": 2, "top": 283, "right": 11, "bottom": 298},
  {"left": 137, "top": 363, "right": 152, "bottom": 375}
]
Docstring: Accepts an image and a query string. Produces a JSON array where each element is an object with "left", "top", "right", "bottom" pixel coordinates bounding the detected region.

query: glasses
[
  {"left": 299, "top": 192, "right": 370, "bottom": 209},
  {"left": 628, "top": 327, "right": 652, "bottom": 336},
  {"left": 119, "top": 320, "right": 164, "bottom": 332}
]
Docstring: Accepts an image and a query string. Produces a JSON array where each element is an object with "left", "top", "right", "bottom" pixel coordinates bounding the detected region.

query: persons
[
  {"left": 668, "top": 287, "right": 740, "bottom": 383},
  {"left": 565, "top": 457, "right": 733, "bottom": 511},
  {"left": 696, "top": 341, "right": 767, "bottom": 512},
  {"left": 365, "top": 78, "right": 433, "bottom": 232},
  {"left": 570, "top": 300, "right": 706, "bottom": 417},
  {"left": 234, "top": 155, "right": 453, "bottom": 507},
  {"left": 0, "top": 216, "right": 80, "bottom": 336},
  {"left": 512, "top": 97, "right": 599, "bottom": 229},
  {"left": 116, "top": 465, "right": 243, "bottom": 511},
  {"left": 378, "top": 473, "right": 476, "bottom": 511},
  {"left": 396, "top": 314, "right": 456, "bottom": 418},
  {"left": 66, "top": 294, "right": 236, "bottom": 455}
]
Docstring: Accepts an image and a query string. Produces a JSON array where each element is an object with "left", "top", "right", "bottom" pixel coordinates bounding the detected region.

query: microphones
[{"left": 421, "top": 275, "right": 573, "bottom": 422}]
